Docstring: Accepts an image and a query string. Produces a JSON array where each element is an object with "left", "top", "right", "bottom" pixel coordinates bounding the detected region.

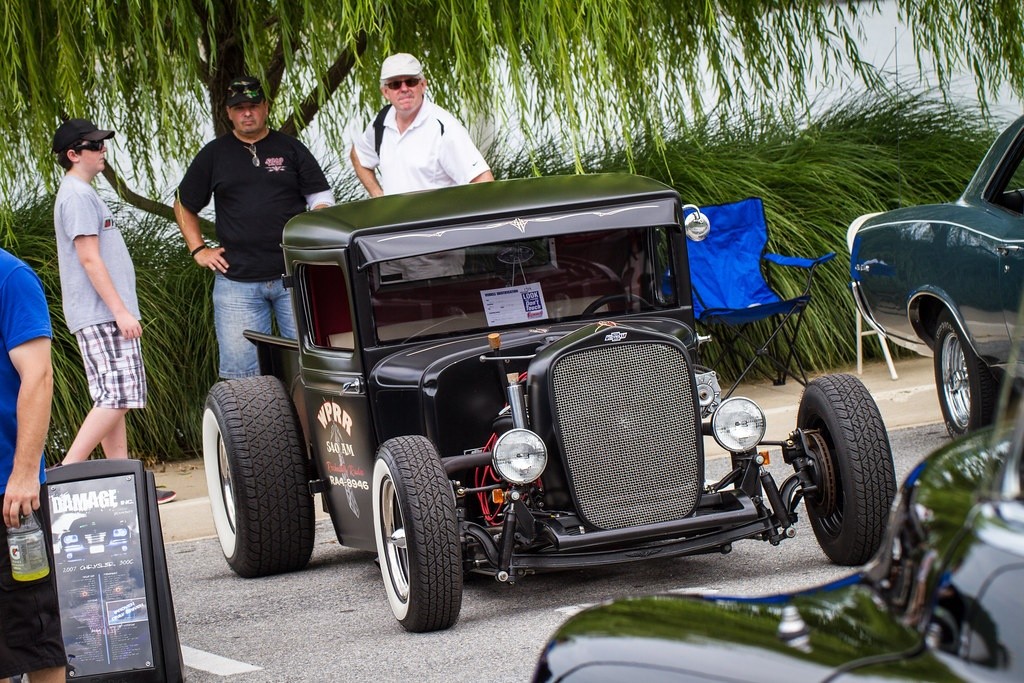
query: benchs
[{"left": 325, "top": 295, "right": 610, "bottom": 349}]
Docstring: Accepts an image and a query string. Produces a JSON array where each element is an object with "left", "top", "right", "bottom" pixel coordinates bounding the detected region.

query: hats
[
  {"left": 225, "top": 76, "right": 265, "bottom": 108},
  {"left": 53, "top": 117, "right": 116, "bottom": 153},
  {"left": 380, "top": 52, "right": 424, "bottom": 83}
]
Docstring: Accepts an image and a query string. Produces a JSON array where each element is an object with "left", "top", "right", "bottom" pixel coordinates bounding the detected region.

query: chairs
[{"left": 661, "top": 196, "right": 838, "bottom": 402}]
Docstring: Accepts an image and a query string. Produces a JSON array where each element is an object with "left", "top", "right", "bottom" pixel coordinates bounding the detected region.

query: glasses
[
  {"left": 245, "top": 143, "right": 260, "bottom": 168},
  {"left": 383, "top": 77, "right": 422, "bottom": 90},
  {"left": 73, "top": 140, "right": 105, "bottom": 151}
]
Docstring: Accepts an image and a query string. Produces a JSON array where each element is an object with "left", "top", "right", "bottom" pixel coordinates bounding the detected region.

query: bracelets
[{"left": 192, "top": 245, "right": 208, "bottom": 258}]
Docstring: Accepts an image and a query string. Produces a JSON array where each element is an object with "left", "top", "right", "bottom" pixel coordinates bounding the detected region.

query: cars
[
  {"left": 844, "top": 116, "right": 1024, "bottom": 444},
  {"left": 201, "top": 172, "right": 902, "bottom": 634},
  {"left": 532, "top": 415, "right": 1024, "bottom": 681}
]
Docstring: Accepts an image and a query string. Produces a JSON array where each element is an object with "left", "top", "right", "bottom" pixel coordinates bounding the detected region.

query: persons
[
  {"left": 350, "top": 53, "right": 493, "bottom": 199},
  {"left": 173, "top": 76, "right": 336, "bottom": 381},
  {"left": 0, "top": 248, "right": 67, "bottom": 683},
  {"left": 45, "top": 118, "right": 175, "bottom": 503}
]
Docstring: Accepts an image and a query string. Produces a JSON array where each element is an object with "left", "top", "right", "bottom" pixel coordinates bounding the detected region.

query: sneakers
[{"left": 155, "top": 489, "right": 176, "bottom": 506}]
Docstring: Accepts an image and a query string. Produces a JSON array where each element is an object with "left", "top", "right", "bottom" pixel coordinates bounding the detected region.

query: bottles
[{"left": 7, "top": 511, "right": 50, "bottom": 581}]
